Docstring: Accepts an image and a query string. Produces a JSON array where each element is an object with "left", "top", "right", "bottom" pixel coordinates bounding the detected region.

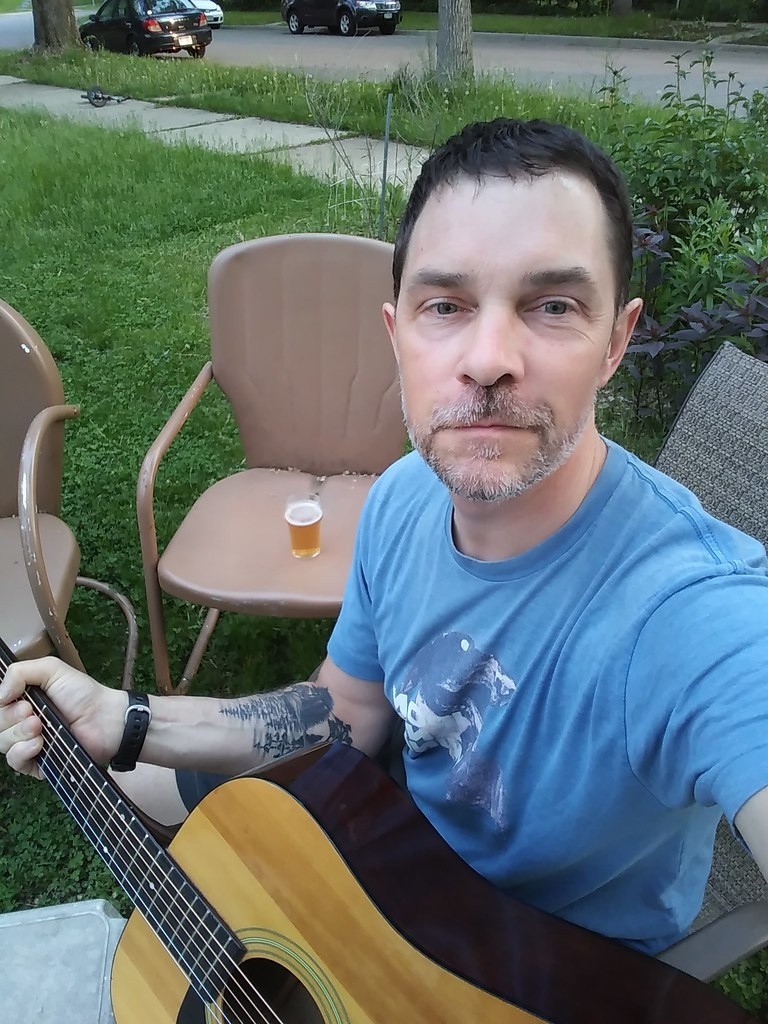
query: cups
[{"left": 286, "top": 502, "right": 323, "bottom": 559}]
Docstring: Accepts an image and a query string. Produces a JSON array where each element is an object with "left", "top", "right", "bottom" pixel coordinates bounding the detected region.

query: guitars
[{"left": 0, "top": 626, "right": 768, "bottom": 1023}]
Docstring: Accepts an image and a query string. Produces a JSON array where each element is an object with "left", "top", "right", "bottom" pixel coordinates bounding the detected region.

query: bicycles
[{"left": 81, "top": 85, "right": 143, "bottom": 106}]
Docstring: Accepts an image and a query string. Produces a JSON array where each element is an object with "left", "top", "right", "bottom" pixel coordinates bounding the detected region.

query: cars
[
  {"left": 191, "top": 0, "right": 224, "bottom": 29},
  {"left": 281, "top": 0, "right": 403, "bottom": 36},
  {"left": 78, "top": 0, "right": 213, "bottom": 58}
]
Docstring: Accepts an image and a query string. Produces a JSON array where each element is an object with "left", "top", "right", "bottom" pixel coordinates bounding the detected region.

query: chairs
[
  {"left": 653, "top": 338, "right": 768, "bottom": 982},
  {"left": 138, "top": 232, "right": 411, "bottom": 697},
  {"left": 1, "top": 302, "right": 139, "bottom": 696}
]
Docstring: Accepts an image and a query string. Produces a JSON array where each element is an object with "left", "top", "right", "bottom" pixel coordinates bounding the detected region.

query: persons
[{"left": 0, "top": 117, "right": 768, "bottom": 1024}]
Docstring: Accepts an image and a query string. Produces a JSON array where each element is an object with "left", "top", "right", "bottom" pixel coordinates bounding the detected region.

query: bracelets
[{"left": 111, "top": 690, "right": 151, "bottom": 774}]
